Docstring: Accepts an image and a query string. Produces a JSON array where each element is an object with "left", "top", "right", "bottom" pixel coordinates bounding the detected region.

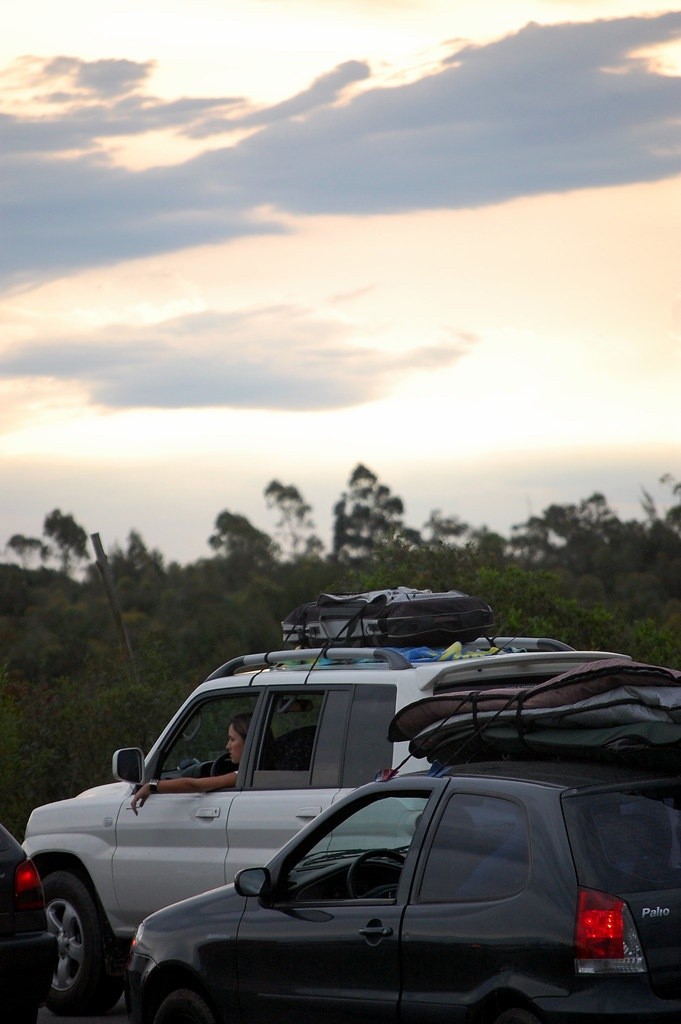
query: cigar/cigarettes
[{"left": 126, "top": 806, "right": 141, "bottom": 810}]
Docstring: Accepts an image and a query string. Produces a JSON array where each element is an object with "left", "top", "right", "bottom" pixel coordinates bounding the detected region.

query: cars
[{"left": 0, "top": 819, "right": 60, "bottom": 1024}]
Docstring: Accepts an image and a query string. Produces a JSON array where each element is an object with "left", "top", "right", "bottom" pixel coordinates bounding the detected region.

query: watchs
[{"left": 149, "top": 778, "right": 159, "bottom": 794}]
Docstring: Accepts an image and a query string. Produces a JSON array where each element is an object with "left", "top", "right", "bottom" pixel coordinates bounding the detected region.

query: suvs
[
  {"left": 21, "top": 637, "right": 632, "bottom": 1017},
  {"left": 123, "top": 760, "right": 681, "bottom": 1024}
]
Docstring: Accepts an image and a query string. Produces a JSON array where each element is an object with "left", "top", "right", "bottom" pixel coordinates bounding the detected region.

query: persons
[
  {"left": 130, "top": 713, "right": 275, "bottom": 816},
  {"left": 416, "top": 808, "right": 474, "bottom": 850}
]
